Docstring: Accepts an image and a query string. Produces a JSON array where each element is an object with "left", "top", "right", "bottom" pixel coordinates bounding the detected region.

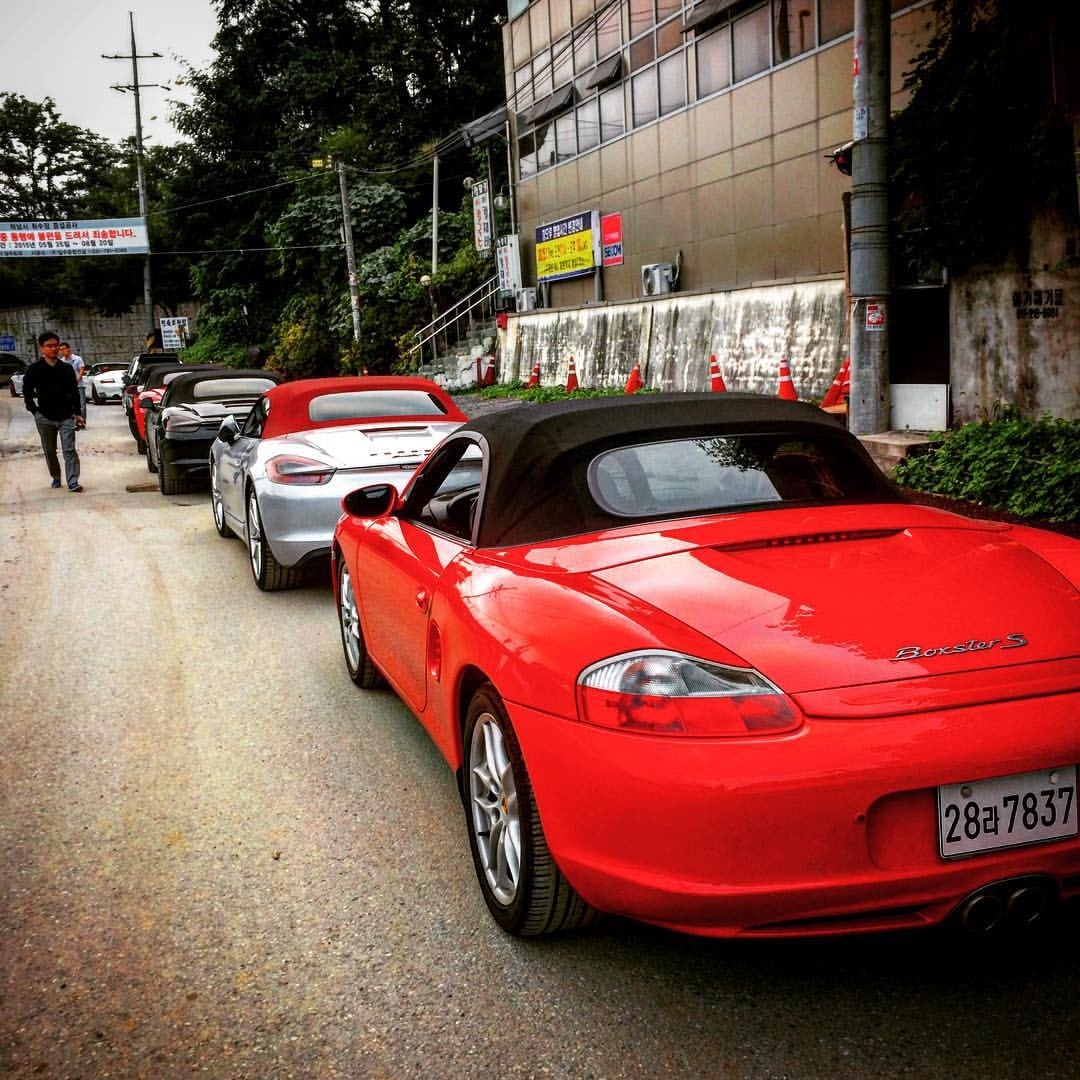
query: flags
[{"left": 471, "top": 178, "right": 492, "bottom": 252}]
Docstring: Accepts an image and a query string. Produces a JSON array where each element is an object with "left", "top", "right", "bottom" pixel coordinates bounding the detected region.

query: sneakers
[
  {"left": 68, "top": 483, "right": 83, "bottom": 492},
  {"left": 52, "top": 479, "right": 62, "bottom": 488}
]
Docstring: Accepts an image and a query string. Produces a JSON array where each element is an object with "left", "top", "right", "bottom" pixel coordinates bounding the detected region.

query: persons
[
  {"left": 22, "top": 330, "right": 86, "bottom": 493},
  {"left": 58, "top": 342, "right": 87, "bottom": 431}
]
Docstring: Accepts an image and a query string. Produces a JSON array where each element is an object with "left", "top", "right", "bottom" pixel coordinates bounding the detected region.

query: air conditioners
[
  {"left": 513, "top": 287, "right": 539, "bottom": 313},
  {"left": 639, "top": 262, "right": 673, "bottom": 299}
]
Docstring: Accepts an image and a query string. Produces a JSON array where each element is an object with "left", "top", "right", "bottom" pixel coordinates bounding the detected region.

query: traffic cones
[
  {"left": 564, "top": 357, "right": 581, "bottom": 394},
  {"left": 710, "top": 356, "right": 726, "bottom": 392},
  {"left": 622, "top": 362, "right": 642, "bottom": 393},
  {"left": 818, "top": 357, "right": 848, "bottom": 412},
  {"left": 777, "top": 356, "right": 810, "bottom": 403},
  {"left": 524, "top": 360, "right": 540, "bottom": 388},
  {"left": 478, "top": 351, "right": 500, "bottom": 387}
]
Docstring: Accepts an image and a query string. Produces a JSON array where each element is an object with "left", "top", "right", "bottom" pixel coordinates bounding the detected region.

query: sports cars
[
  {"left": 139, "top": 371, "right": 276, "bottom": 497},
  {"left": 82, "top": 361, "right": 129, "bottom": 405},
  {"left": 209, "top": 374, "right": 471, "bottom": 592},
  {"left": 125, "top": 351, "right": 217, "bottom": 452},
  {"left": 328, "top": 392, "right": 1079, "bottom": 945},
  {"left": 10, "top": 368, "right": 38, "bottom": 398}
]
